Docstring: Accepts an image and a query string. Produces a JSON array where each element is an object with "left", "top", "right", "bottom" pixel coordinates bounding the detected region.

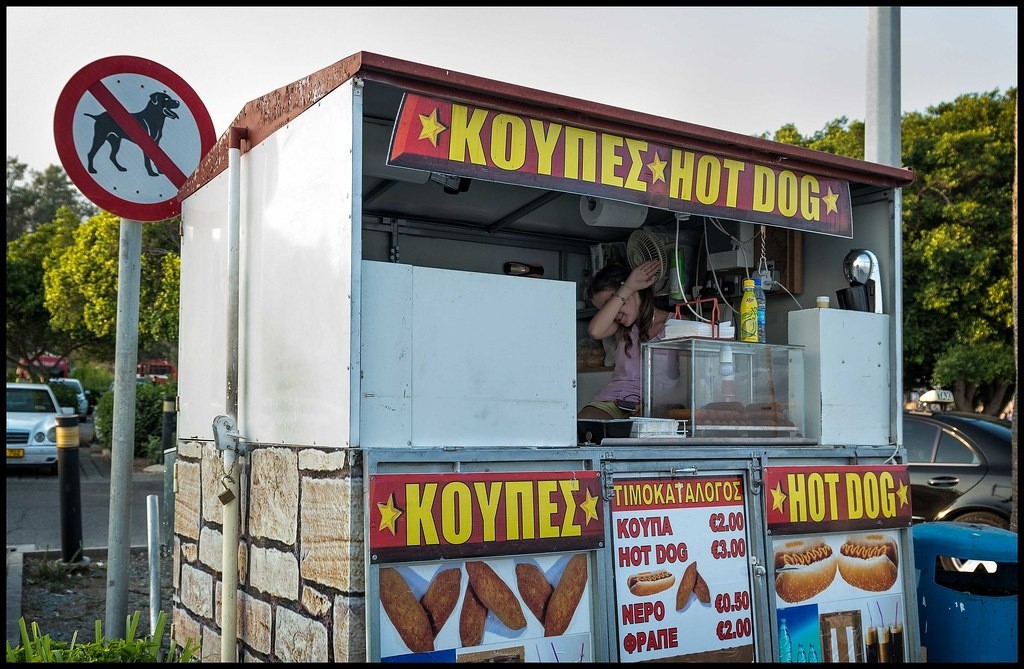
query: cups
[
  {"left": 821, "top": 609, "right": 864, "bottom": 662},
  {"left": 817, "top": 297, "right": 832, "bottom": 309},
  {"left": 864, "top": 624, "right": 905, "bottom": 663}
]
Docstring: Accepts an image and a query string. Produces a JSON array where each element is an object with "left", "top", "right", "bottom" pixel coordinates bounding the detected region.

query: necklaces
[{"left": 650, "top": 307, "right": 655, "bottom": 328}]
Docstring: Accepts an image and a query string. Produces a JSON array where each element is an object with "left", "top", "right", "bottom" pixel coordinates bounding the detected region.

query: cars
[
  {"left": 6, "top": 383, "right": 77, "bottom": 475},
  {"left": 49, "top": 378, "right": 92, "bottom": 416},
  {"left": 902, "top": 390, "right": 1017, "bottom": 530}
]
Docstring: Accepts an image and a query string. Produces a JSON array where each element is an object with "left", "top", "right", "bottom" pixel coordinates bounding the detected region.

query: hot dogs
[
  {"left": 837, "top": 531, "right": 899, "bottom": 592},
  {"left": 771, "top": 537, "right": 836, "bottom": 603},
  {"left": 628, "top": 569, "right": 676, "bottom": 596}
]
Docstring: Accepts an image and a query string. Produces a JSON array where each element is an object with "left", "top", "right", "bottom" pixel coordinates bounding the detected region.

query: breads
[{"left": 576, "top": 343, "right": 606, "bottom": 369}]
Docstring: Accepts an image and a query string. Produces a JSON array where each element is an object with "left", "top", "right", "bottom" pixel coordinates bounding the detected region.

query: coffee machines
[{"left": 836, "top": 248, "right": 883, "bottom": 314}]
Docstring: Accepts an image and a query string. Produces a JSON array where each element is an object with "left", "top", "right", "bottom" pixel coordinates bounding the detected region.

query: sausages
[{"left": 668, "top": 401, "right": 796, "bottom": 427}]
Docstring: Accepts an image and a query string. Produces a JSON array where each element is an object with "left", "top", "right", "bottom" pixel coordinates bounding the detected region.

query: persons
[
  {"left": 1004, "top": 412, "right": 1013, "bottom": 420},
  {"left": 577, "top": 259, "right": 690, "bottom": 420},
  {"left": 152, "top": 376, "right": 157, "bottom": 382},
  {"left": 932, "top": 404, "right": 938, "bottom": 410}
]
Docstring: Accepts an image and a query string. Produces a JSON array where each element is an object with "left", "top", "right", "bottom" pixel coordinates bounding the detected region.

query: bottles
[
  {"left": 809, "top": 642, "right": 820, "bottom": 663},
  {"left": 502, "top": 262, "right": 545, "bottom": 276},
  {"left": 740, "top": 280, "right": 758, "bottom": 343},
  {"left": 753, "top": 278, "right": 767, "bottom": 342},
  {"left": 779, "top": 619, "right": 792, "bottom": 663},
  {"left": 797, "top": 642, "right": 807, "bottom": 663}
]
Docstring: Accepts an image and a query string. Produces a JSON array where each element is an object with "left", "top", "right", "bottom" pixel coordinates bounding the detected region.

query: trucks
[
  {"left": 137, "top": 359, "right": 178, "bottom": 384},
  {"left": 14, "top": 355, "right": 70, "bottom": 383}
]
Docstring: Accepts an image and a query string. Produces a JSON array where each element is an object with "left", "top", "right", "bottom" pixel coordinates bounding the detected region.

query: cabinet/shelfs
[{"left": 636, "top": 339, "right": 806, "bottom": 441}]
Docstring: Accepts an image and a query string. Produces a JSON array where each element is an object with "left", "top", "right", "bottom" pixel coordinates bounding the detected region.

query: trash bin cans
[{"left": 912, "top": 521, "right": 1018, "bottom": 663}]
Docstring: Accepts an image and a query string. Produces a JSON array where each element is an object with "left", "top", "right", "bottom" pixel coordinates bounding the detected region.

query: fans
[{"left": 624, "top": 230, "right": 674, "bottom": 287}]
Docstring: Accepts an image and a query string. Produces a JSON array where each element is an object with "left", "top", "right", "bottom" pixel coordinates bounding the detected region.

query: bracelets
[
  {"left": 611, "top": 293, "right": 625, "bottom": 306},
  {"left": 621, "top": 282, "right": 636, "bottom": 293}
]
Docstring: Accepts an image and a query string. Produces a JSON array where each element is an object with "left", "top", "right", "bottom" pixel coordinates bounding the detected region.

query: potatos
[
  {"left": 675, "top": 561, "right": 712, "bottom": 610},
  {"left": 380, "top": 553, "right": 587, "bottom": 652}
]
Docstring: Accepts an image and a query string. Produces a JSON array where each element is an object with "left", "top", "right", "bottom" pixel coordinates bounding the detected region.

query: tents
[{"left": 19, "top": 349, "right": 69, "bottom": 379}]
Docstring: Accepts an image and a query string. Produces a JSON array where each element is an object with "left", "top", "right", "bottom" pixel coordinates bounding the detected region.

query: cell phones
[{"left": 614, "top": 399, "right": 637, "bottom": 412}]
